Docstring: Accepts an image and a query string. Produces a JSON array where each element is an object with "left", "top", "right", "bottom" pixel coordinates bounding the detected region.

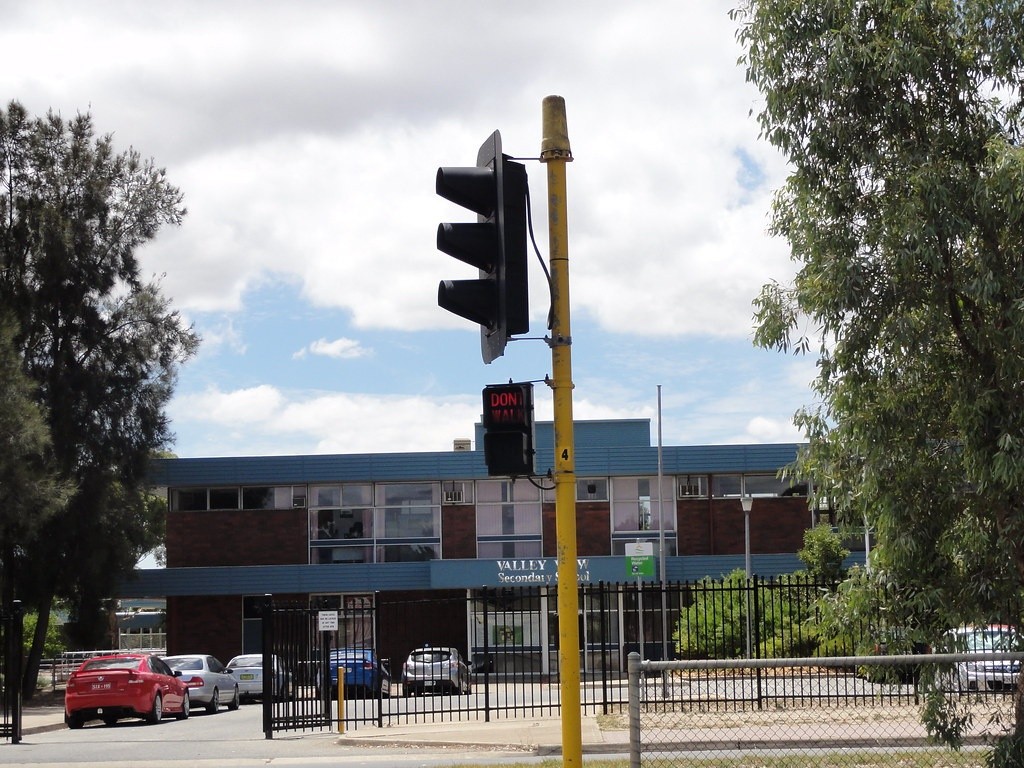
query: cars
[
  {"left": 224, "top": 653, "right": 293, "bottom": 703},
  {"left": 857, "top": 629, "right": 933, "bottom": 686},
  {"left": 402, "top": 649, "right": 473, "bottom": 697},
  {"left": 316, "top": 647, "right": 392, "bottom": 697},
  {"left": 933, "top": 625, "right": 1021, "bottom": 693},
  {"left": 65, "top": 653, "right": 191, "bottom": 729},
  {"left": 160, "top": 654, "right": 240, "bottom": 713}
]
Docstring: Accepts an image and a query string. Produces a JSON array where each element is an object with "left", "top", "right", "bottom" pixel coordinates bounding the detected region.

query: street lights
[{"left": 740, "top": 494, "right": 753, "bottom": 657}]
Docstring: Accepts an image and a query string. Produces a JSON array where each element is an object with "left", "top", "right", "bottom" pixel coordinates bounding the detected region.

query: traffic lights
[
  {"left": 436, "top": 129, "right": 526, "bottom": 363},
  {"left": 478, "top": 386, "right": 539, "bottom": 477}
]
